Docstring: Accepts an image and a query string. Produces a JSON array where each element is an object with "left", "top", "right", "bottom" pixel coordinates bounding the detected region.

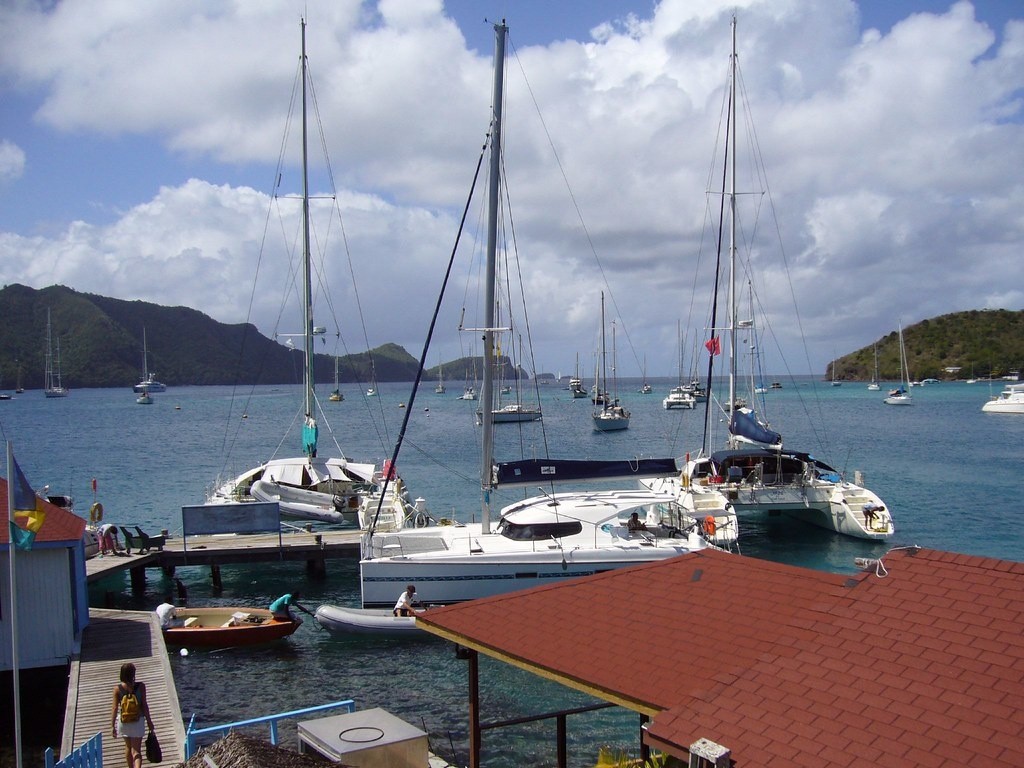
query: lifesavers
[
  {"left": 681, "top": 473, "right": 689, "bottom": 487},
  {"left": 704, "top": 516, "right": 716, "bottom": 534},
  {"left": 91, "top": 502, "right": 103, "bottom": 521}
]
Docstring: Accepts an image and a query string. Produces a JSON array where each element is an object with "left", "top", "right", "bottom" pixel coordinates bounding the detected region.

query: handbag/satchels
[{"left": 145, "top": 729, "right": 162, "bottom": 763}]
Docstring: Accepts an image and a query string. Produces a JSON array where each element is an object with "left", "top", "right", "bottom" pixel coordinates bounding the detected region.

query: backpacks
[{"left": 118, "top": 682, "right": 142, "bottom": 723}]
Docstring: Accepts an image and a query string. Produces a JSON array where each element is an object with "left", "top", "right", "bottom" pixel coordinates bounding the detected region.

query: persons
[
  {"left": 156, "top": 596, "right": 184, "bottom": 629},
  {"left": 269, "top": 589, "right": 308, "bottom": 622},
  {"left": 862, "top": 502, "right": 884, "bottom": 531},
  {"left": 111, "top": 663, "right": 154, "bottom": 768},
  {"left": 627, "top": 513, "right": 647, "bottom": 532},
  {"left": 512, "top": 525, "right": 531, "bottom": 538},
  {"left": 393, "top": 585, "right": 420, "bottom": 617},
  {"left": 97, "top": 523, "right": 118, "bottom": 555}
]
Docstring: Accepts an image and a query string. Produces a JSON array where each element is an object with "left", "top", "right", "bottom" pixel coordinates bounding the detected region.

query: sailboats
[
  {"left": 206, "top": 19, "right": 430, "bottom": 534},
  {"left": 134, "top": 325, "right": 166, "bottom": 392},
  {"left": 640, "top": 19, "right": 896, "bottom": 540},
  {"left": 43, "top": 307, "right": 69, "bottom": 397},
  {"left": 326, "top": 284, "right": 1024, "bottom": 430},
  {"left": 362, "top": 19, "right": 728, "bottom": 597}
]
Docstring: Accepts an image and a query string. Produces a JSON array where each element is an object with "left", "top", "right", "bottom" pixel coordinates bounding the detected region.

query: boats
[
  {"left": 314, "top": 602, "right": 448, "bottom": 639},
  {"left": 158, "top": 607, "right": 304, "bottom": 644},
  {"left": 0, "top": 384, "right": 25, "bottom": 399},
  {"left": 136, "top": 387, "right": 154, "bottom": 404}
]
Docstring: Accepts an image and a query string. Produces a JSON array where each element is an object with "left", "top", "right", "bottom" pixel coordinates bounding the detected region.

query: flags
[
  {"left": 9, "top": 455, "right": 46, "bottom": 551},
  {"left": 705, "top": 335, "right": 720, "bottom": 356}
]
